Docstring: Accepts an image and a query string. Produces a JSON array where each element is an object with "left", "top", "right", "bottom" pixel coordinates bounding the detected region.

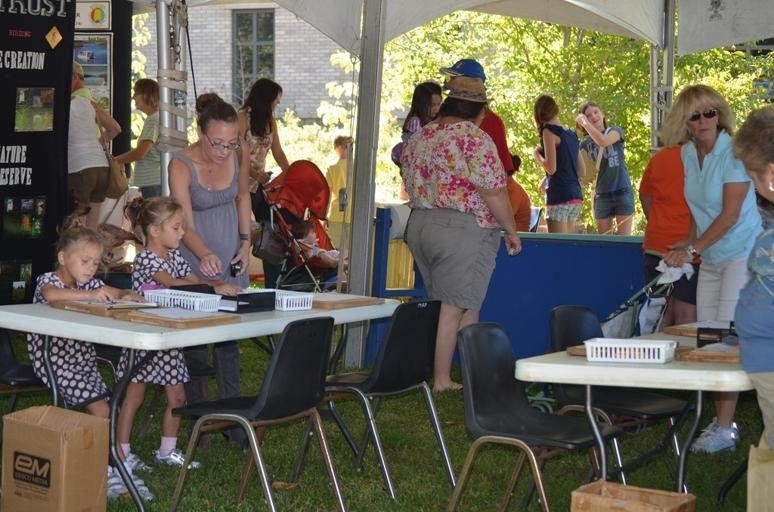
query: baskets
[
  {"left": 244, "top": 287, "right": 314, "bottom": 311},
  {"left": 146, "top": 286, "right": 221, "bottom": 311},
  {"left": 584, "top": 337, "right": 678, "bottom": 364}
]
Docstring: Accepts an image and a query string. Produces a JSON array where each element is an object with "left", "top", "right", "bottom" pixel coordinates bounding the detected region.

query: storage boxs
[
  {"left": 570, "top": 480, "right": 696, "bottom": 512},
  {"left": 2, "top": 406, "right": 110, "bottom": 512}
]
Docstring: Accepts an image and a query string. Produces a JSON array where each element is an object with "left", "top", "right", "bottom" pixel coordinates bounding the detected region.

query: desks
[
  {"left": 515, "top": 320, "right": 755, "bottom": 504},
  {"left": 0, "top": 287, "right": 401, "bottom": 512}
]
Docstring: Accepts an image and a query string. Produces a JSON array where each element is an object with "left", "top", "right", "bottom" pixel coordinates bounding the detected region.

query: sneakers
[
  {"left": 691, "top": 415, "right": 740, "bottom": 455},
  {"left": 107, "top": 445, "right": 200, "bottom": 502},
  {"left": 205, "top": 132, "right": 240, "bottom": 151}
]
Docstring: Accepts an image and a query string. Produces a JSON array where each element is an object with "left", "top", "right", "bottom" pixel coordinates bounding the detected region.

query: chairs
[
  {"left": 521, "top": 305, "right": 687, "bottom": 512},
  {"left": 0, "top": 328, "right": 58, "bottom": 413},
  {"left": 292, "top": 299, "right": 457, "bottom": 499},
  {"left": 447, "top": 322, "right": 627, "bottom": 512},
  {"left": 170, "top": 317, "right": 348, "bottom": 512},
  {"left": 93, "top": 345, "right": 217, "bottom": 447},
  {"left": 529, "top": 207, "right": 543, "bottom": 232}
]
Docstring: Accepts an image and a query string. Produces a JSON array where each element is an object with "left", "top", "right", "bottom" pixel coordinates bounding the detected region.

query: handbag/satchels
[
  {"left": 107, "top": 157, "right": 128, "bottom": 198},
  {"left": 252, "top": 220, "right": 292, "bottom": 265}
]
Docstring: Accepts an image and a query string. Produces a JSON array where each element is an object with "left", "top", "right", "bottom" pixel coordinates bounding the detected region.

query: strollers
[
  {"left": 257, "top": 160, "right": 350, "bottom": 298},
  {"left": 524, "top": 273, "right": 673, "bottom": 434}
]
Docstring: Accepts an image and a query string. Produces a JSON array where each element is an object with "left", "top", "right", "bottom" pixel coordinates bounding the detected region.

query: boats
[{"left": 75, "top": 50, "right": 95, "bottom": 62}]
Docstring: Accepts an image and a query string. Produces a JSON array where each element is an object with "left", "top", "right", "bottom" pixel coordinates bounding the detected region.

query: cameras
[{"left": 231, "top": 254, "right": 240, "bottom": 277}]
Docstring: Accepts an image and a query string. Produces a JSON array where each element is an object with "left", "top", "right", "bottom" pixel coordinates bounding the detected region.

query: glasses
[{"left": 690, "top": 108, "right": 720, "bottom": 121}]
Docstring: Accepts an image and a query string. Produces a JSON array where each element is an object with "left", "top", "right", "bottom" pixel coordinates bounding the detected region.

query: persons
[
  {"left": 234, "top": 77, "right": 353, "bottom": 270},
  {"left": 25, "top": 216, "right": 155, "bottom": 501},
  {"left": 639, "top": 85, "right": 772, "bottom": 454},
  {"left": 576, "top": 102, "right": 635, "bottom": 235},
  {"left": 70, "top": 63, "right": 162, "bottom": 231},
  {"left": 532, "top": 94, "right": 587, "bottom": 234},
  {"left": 115, "top": 196, "right": 201, "bottom": 472},
  {"left": 394, "top": 60, "right": 530, "bottom": 391},
  {"left": 169, "top": 93, "right": 251, "bottom": 448}
]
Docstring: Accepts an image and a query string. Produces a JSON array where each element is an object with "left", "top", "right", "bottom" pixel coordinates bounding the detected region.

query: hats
[{"left": 440, "top": 58, "right": 491, "bottom": 102}]
